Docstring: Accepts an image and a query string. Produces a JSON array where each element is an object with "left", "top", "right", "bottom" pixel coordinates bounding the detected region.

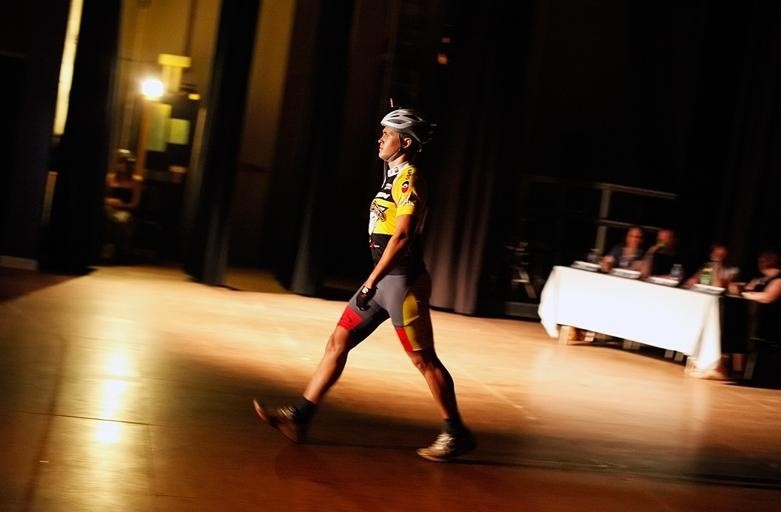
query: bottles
[
  {"left": 670, "top": 263, "right": 684, "bottom": 277},
  {"left": 701, "top": 269, "right": 713, "bottom": 284}
]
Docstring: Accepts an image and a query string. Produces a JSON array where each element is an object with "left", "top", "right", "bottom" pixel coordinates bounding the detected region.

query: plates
[
  {"left": 576, "top": 260, "right": 601, "bottom": 272},
  {"left": 648, "top": 275, "right": 680, "bottom": 286},
  {"left": 611, "top": 267, "right": 641, "bottom": 280},
  {"left": 693, "top": 283, "right": 726, "bottom": 295}
]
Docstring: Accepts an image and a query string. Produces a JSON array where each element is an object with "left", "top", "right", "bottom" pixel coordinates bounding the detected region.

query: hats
[{"left": 116, "top": 148, "right": 132, "bottom": 159}]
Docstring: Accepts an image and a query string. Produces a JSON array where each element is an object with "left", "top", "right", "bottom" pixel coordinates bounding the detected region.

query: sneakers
[
  {"left": 416, "top": 431, "right": 480, "bottom": 463},
  {"left": 252, "top": 396, "right": 313, "bottom": 444}
]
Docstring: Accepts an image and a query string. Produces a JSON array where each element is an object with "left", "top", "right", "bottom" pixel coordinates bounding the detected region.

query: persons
[
  {"left": 729, "top": 246, "right": 780, "bottom": 305},
  {"left": 639, "top": 228, "right": 686, "bottom": 279},
  {"left": 253, "top": 110, "right": 473, "bottom": 463},
  {"left": 598, "top": 223, "right": 644, "bottom": 267},
  {"left": 103, "top": 147, "right": 144, "bottom": 254},
  {"left": 684, "top": 244, "right": 737, "bottom": 289}
]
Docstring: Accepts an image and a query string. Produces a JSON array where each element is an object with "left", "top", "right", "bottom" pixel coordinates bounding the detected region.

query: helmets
[{"left": 380, "top": 108, "right": 437, "bottom": 151}]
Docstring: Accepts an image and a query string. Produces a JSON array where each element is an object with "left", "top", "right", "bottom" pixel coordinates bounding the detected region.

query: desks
[{"left": 549, "top": 264, "right": 756, "bottom": 375}]
